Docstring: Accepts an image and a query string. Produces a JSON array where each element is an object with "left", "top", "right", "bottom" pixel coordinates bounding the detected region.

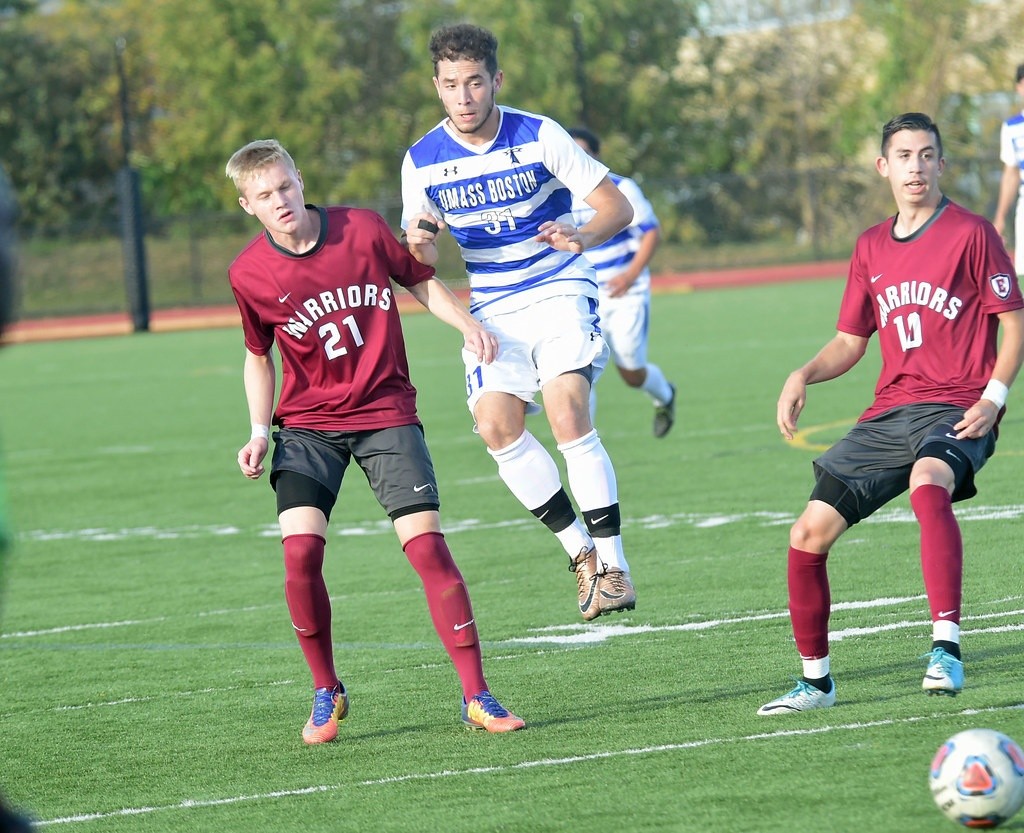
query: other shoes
[{"left": 655, "top": 382, "right": 675, "bottom": 438}]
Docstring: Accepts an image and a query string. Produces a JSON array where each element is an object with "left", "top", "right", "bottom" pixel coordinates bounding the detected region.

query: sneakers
[
  {"left": 918, "top": 647, "right": 963, "bottom": 698},
  {"left": 588, "top": 554, "right": 636, "bottom": 616},
  {"left": 302, "top": 680, "right": 349, "bottom": 744},
  {"left": 568, "top": 532, "right": 602, "bottom": 621},
  {"left": 461, "top": 691, "right": 525, "bottom": 734},
  {"left": 756, "top": 677, "right": 835, "bottom": 715}
]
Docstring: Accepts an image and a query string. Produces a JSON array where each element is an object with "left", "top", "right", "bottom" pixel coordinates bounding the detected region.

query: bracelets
[{"left": 980, "top": 378, "right": 1009, "bottom": 411}]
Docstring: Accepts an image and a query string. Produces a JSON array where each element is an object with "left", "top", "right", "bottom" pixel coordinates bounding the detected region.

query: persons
[
  {"left": 224, "top": 139, "right": 527, "bottom": 745},
  {"left": 756, "top": 113, "right": 1024, "bottom": 717},
  {"left": 566, "top": 128, "right": 678, "bottom": 441},
  {"left": 991, "top": 63, "right": 1024, "bottom": 272},
  {"left": 400, "top": 24, "right": 636, "bottom": 622}
]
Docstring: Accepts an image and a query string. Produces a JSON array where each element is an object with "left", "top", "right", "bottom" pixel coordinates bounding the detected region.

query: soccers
[{"left": 934, "top": 729, "right": 1024, "bottom": 831}]
